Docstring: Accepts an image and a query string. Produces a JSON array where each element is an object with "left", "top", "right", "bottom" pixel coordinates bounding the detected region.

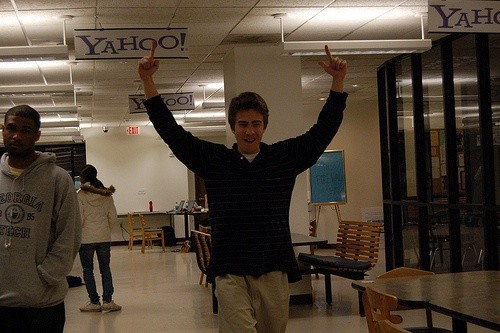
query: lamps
[{"left": 0, "top": 0, "right": 432, "bottom": 147}]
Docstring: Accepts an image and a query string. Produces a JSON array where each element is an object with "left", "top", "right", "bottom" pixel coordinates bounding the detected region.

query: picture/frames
[{"left": 309, "top": 148, "right": 347, "bottom": 205}]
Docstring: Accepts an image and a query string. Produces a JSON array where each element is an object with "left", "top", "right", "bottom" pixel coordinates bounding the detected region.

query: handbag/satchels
[{"left": 66, "top": 275, "right": 85, "bottom": 287}]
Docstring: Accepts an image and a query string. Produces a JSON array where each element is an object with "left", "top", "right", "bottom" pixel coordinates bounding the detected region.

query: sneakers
[
  {"left": 80, "top": 301, "right": 102, "bottom": 312},
  {"left": 102, "top": 300, "right": 121, "bottom": 311}
]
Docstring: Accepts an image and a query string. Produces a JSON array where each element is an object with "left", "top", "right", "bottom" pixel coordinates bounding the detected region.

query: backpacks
[{"left": 157, "top": 225, "right": 177, "bottom": 246}]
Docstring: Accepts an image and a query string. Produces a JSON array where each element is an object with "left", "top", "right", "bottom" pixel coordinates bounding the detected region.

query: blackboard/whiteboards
[{"left": 310, "top": 149, "right": 347, "bottom": 205}]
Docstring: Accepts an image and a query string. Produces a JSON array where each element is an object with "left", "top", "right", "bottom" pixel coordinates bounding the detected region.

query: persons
[
  {"left": 0, "top": 104, "right": 82, "bottom": 333},
  {"left": 76, "top": 164, "right": 121, "bottom": 312},
  {"left": 138, "top": 42, "right": 348, "bottom": 333}
]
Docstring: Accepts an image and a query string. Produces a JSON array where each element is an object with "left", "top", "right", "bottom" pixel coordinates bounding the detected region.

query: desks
[
  {"left": 116, "top": 210, "right": 166, "bottom": 218},
  {"left": 167, "top": 208, "right": 208, "bottom": 252},
  {"left": 291, "top": 232, "right": 328, "bottom": 247},
  {"left": 352, "top": 270, "right": 500, "bottom": 333}
]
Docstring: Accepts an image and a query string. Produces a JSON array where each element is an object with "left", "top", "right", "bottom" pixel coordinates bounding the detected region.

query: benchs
[
  {"left": 191, "top": 229, "right": 219, "bottom": 314},
  {"left": 298, "top": 220, "right": 383, "bottom": 316}
]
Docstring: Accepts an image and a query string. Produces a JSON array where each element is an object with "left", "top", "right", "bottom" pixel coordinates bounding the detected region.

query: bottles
[
  {"left": 149, "top": 200, "right": 153, "bottom": 212},
  {"left": 175, "top": 202, "right": 179, "bottom": 214}
]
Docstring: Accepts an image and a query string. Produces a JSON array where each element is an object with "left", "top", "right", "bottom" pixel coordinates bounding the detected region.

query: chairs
[
  {"left": 139, "top": 214, "right": 165, "bottom": 254},
  {"left": 128, "top": 212, "right": 152, "bottom": 250},
  {"left": 377, "top": 267, "right": 452, "bottom": 333},
  {"left": 309, "top": 220, "right": 320, "bottom": 280},
  {"left": 362, "top": 286, "right": 412, "bottom": 333}
]
